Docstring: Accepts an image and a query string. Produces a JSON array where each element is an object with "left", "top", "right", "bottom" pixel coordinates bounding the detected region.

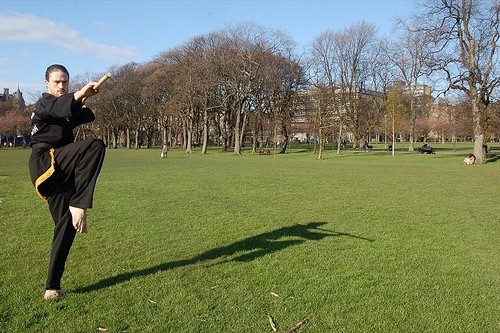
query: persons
[
  {"left": 259, "top": 149, "right": 270, "bottom": 155},
  {"left": 3, "top": 142, "right": 13, "bottom": 147},
  {"left": 343, "top": 140, "right": 346, "bottom": 150},
  {"left": 363, "top": 141, "right": 368, "bottom": 151},
  {"left": 163, "top": 143, "right": 168, "bottom": 158},
  {"left": 464, "top": 153, "right": 476, "bottom": 165},
  {"left": 29, "top": 64, "right": 106, "bottom": 300}
]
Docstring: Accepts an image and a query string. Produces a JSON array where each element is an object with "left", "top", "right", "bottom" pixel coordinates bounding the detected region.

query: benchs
[
  {"left": 361, "top": 145, "right": 373, "bottom": 150},
  {"left": 489, "top": 146, "right": 500, "bottom": 153},
  {"left": 418, "top": 146, "right": 435, "bottom": 154}
]
